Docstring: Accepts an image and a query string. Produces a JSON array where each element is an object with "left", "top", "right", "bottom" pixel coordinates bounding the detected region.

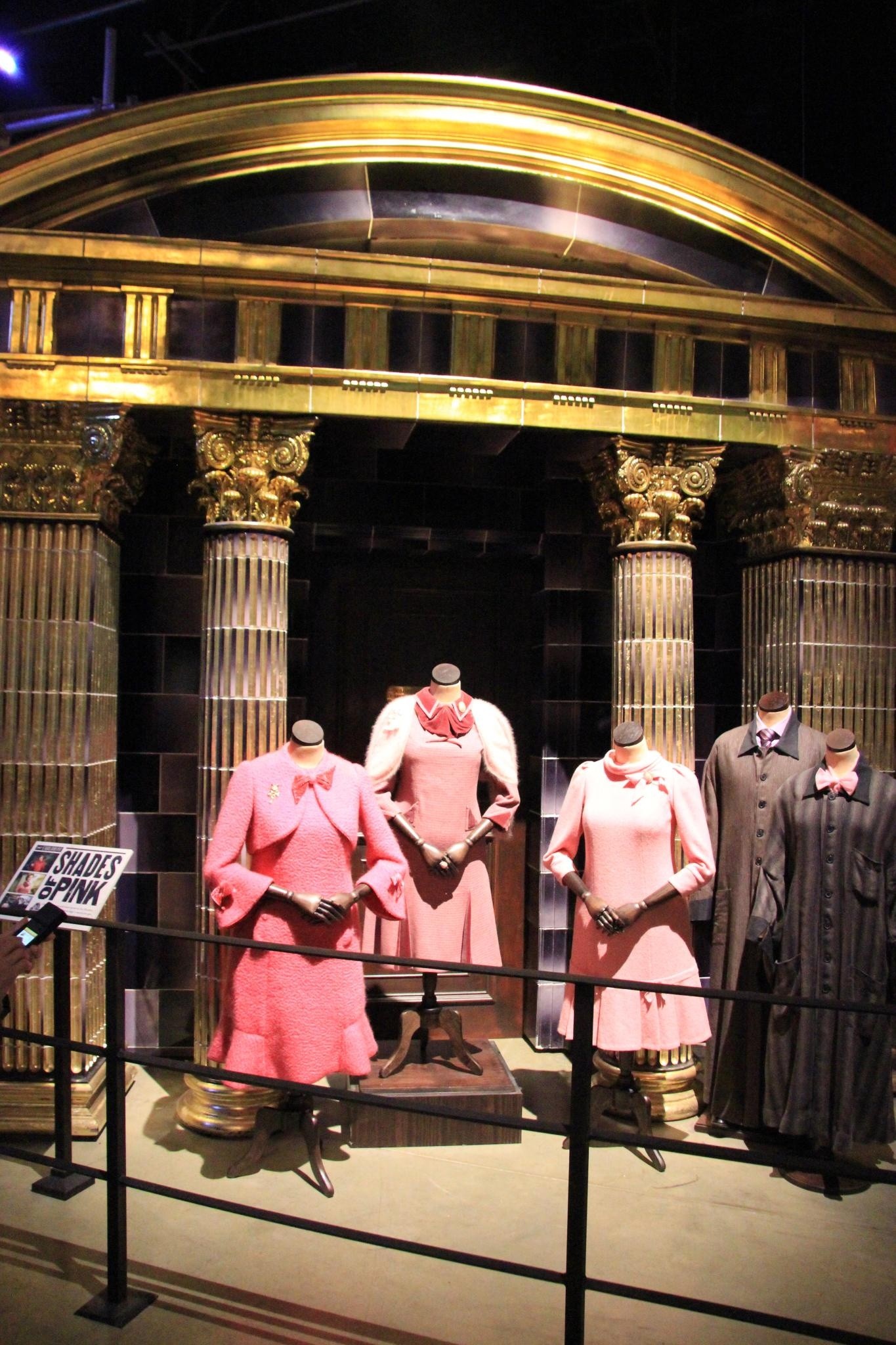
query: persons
[
  {"left": 201, "top": 719, "right": 410, "bottom": 1090},
  {"left": 701, "top": 693, "right": 896, "bottom": 1194},
  {"left": 0, "top": 916, "right": 55, "bottom": 1019},
  {"left": 364, "top": 664, "right": 521, "bottom": 971},
  {"left": 542, "top": 720, "right": 715, "bottom": 1050}
]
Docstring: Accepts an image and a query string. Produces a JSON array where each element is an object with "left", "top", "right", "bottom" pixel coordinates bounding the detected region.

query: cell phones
[{"left": 13, "top": 900, "right": 67, "bottom": 950}]
